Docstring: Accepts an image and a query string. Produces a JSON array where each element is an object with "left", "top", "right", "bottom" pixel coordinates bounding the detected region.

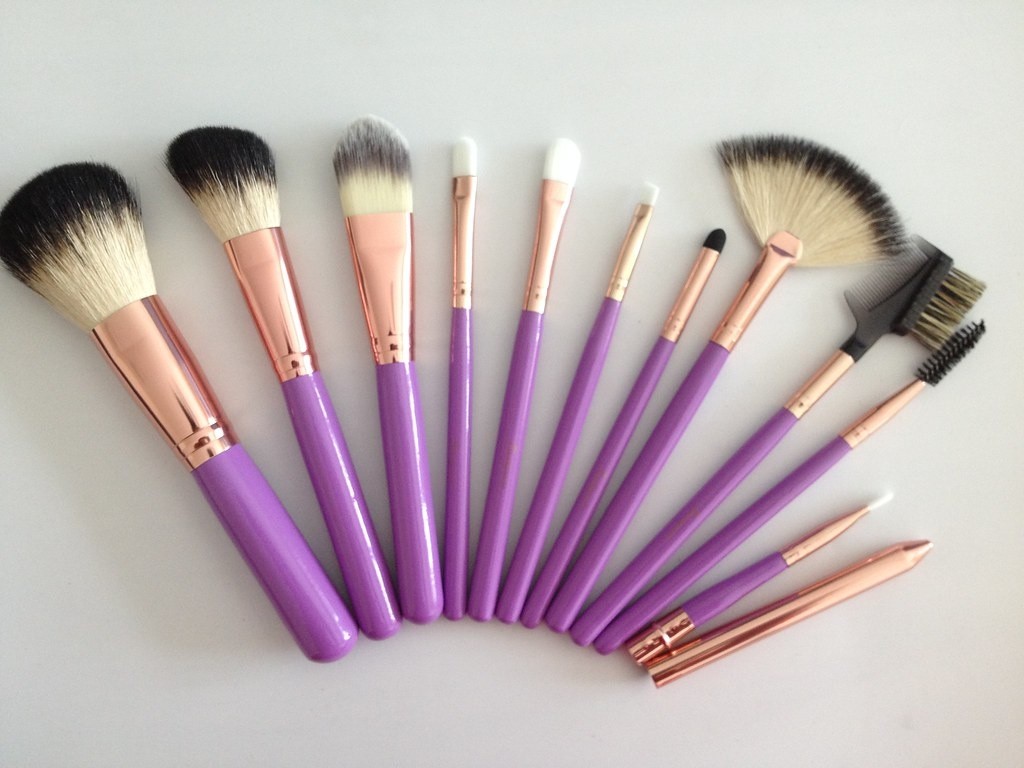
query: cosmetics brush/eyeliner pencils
[{"left": 0, "top": 116, "right": 987, "bottom": 687}]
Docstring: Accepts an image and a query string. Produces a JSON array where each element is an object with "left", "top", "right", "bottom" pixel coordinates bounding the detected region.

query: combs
[{"left": 568, "top": 234, "right": 987, "bottom": 646}]
